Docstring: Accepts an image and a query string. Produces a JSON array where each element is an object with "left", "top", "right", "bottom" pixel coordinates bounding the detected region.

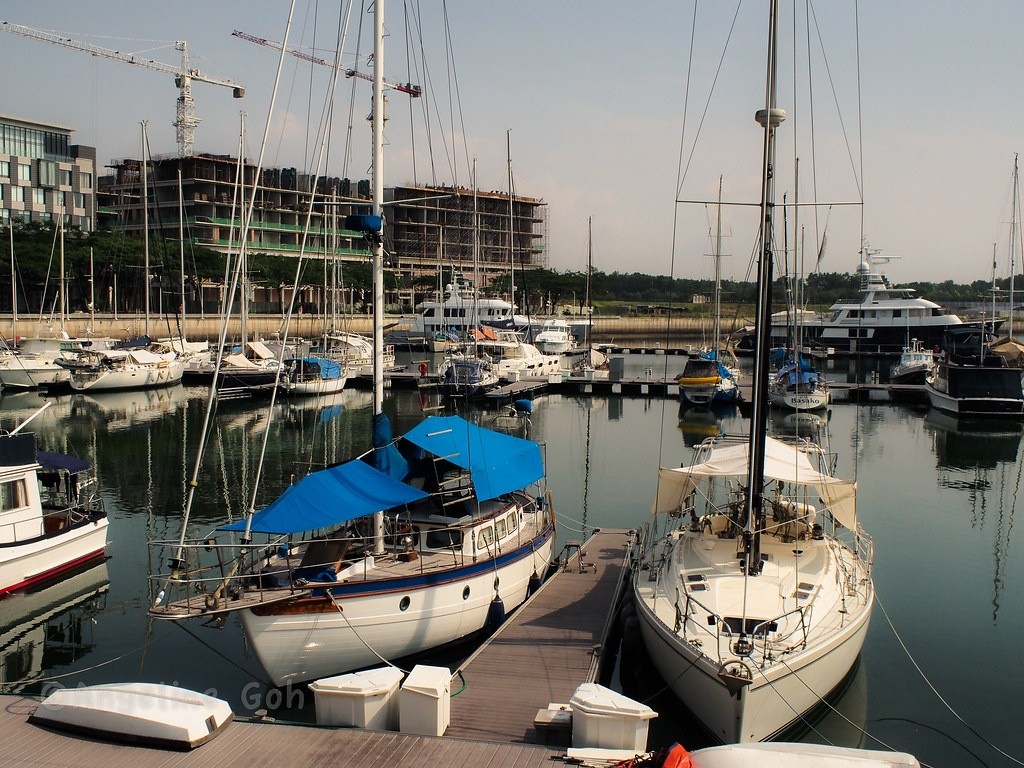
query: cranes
[
  {"left": 0, "top": 21, "right": 245, "bottom": 159},
  {"left": 232, "top": 28, "right": 422, "bottom": 197}
]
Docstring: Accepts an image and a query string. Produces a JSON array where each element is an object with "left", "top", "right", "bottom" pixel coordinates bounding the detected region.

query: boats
[
  {"left": 0, "top": 332, "right": 110, "bottom": 652},
  {"left": 732, "top": 230, "right": 1008, "bottom": 367}
]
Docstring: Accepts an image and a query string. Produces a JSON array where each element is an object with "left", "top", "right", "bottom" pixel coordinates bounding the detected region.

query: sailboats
[
  {"left": 673, "top": 174, "right": 740, "bottom": 402},
  {"left": 889, "top": 152, "right": 1024, "bottom": 430},
  {"left": 776, "top": 159, "right": 830, "bottom": 409},
  {"left": 630, "top": 0, "right": 875, "bottom": 748},
  {"left": 0, "top": 108, "right": 608, "bottom": 397},
  {"left": 147, "top": 0, "right": 553, "bottom": 687}
]
